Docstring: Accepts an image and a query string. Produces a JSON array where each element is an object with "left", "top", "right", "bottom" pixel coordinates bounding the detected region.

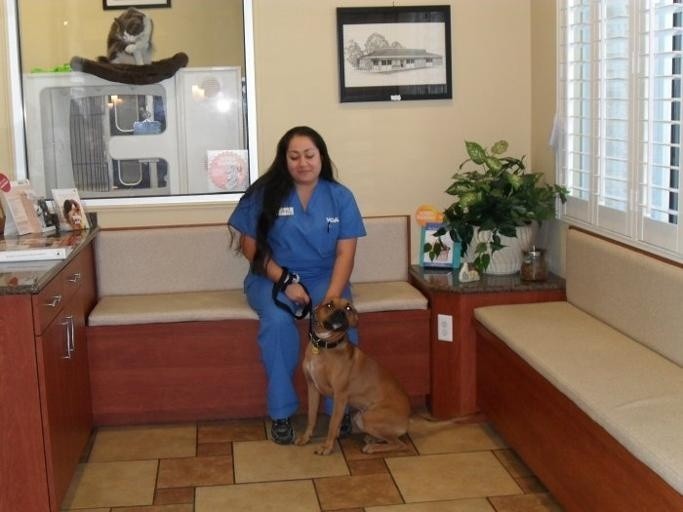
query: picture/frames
[
  {"left": 336, "top": 5, "right": 452, "bottom": 103},
  {"left": 419, "top": 223, "right": 462, "bottom": 268},
  {"left": 102, "top": 0, "right": 171, "bottom": 10}
]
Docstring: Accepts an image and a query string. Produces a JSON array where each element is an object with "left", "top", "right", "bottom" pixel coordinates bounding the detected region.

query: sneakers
[
  {"left": 338, "top": 414, "right": 352, "bottom": 438},
  {"left": 271, "top": 418, "right": 293, "bottom": 444}
]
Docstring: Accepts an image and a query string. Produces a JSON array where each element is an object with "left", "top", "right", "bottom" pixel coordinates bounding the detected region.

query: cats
[{"left": 95, "top": 7, "right": 156, "bottom": 68}]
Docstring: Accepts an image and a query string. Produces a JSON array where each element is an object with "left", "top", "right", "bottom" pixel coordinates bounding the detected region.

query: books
[{"left": 0, "top": 234, "right": 80, "bottom": 263}]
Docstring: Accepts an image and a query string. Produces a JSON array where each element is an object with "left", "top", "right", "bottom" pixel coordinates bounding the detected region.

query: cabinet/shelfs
[{"left": 0, "top": 229, "right": 96, "bottom": 511}]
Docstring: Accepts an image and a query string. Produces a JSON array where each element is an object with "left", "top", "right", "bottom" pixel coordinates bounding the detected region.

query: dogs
[{"left": 291, "top": 296, "right": 482, "bottom": 456}]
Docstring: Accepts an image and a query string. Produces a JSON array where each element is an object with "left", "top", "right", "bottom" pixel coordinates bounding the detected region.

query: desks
[{"left": 407, "top": 261, "right": 562, "bottom": 419}]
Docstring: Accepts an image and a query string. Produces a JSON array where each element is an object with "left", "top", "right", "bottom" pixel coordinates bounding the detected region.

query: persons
[{"left": 228, "top": 126, "right": 367, "bottom": 444}]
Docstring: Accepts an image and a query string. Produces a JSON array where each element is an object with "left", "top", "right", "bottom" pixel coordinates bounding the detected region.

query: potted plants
[{"left": 438, "top": 138, "right": 570, "bottom": 276}]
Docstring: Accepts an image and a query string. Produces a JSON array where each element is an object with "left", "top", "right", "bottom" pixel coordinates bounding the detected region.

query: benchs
[
  {"left": 471, "top": 224, "right": 683, "bottom": 512},
  {"left": 88, "top": 216, "right": 430, "bottom": 423}
]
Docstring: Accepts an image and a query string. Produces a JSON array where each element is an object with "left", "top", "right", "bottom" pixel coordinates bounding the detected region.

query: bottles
[{"left": 520, "top": 247, "right": 550, "bottom": 282}]
